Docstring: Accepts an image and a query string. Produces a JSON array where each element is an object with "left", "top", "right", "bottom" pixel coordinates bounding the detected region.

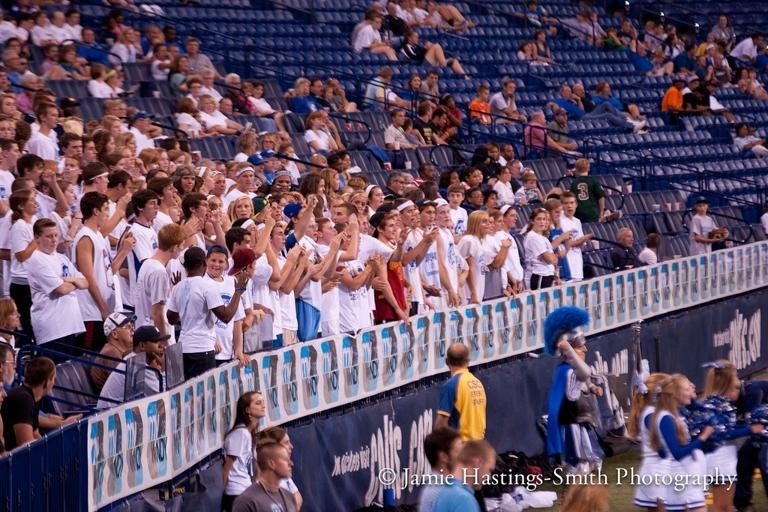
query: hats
[
  {"left": 133, "top": 326, "right": 172, "bottom": 347},
  {"left": 172, "top": 147, "right": 449, "bottom": 279},
  {"left": 694, "top": 196, "right": 711, "bottom": 205},
  {"left": 103, "top": 311, "right": 138, "bottom": 337},
  {"left": 554, "top": 108, "right": 568, "bottom": 115},
  {"left": 687, "top": 75, "right": 699, "bottom": 83},
  {"left": 58, "top": 95, "right": 83, "bottom": 108},
  {"left": 131, "top": 110, "right": 156, "bottom": 121}
]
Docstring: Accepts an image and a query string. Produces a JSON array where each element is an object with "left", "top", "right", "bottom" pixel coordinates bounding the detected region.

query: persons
[
  {"left": 231, "top": 442, "right": 298, "bottom": 512},
  {"left": 219, "top": 391, "right": 266, "bottom": 511},
  {"left": 1, "top": 0, "right": 768, "bottom": 458},
  {"left": 255, "top": 427, "right": 304, "bottom": 512},
  {"left": 412, "top": 458, "right": 767, "bottom": 512}
]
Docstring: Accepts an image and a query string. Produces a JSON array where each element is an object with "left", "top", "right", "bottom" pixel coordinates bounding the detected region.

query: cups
[
  {"left": 605, "top": 184, "right": 633, "bottom": 196},
  {"left": 590, "top": 240, "right": 599, "bottom": 250},
  {"left": 652, "top": 204, "right": 660, "bottom": 213},
  {"left": 404, "top": 161, "right": 412, "bottom": 170},
  {"left": 191, "top": 151, "right": 201, "bottom": 161},
  {"left": 663, "top": 203, "right": 671, "bottom": 212},
  {"left": 153, "top": 91, "right": 160, "bottom": 99},
  {"left": 623, "top": 265, "right": 634, "bottom": 270},
  {"left": 673, "top": 202, "right": 680, "bottom": 211},
  {"left": 393, "top": 141, "right": 400, "bottom": 150},
  {"left": 384, "top": 162, "right": 391, "bottom": 171}
]
